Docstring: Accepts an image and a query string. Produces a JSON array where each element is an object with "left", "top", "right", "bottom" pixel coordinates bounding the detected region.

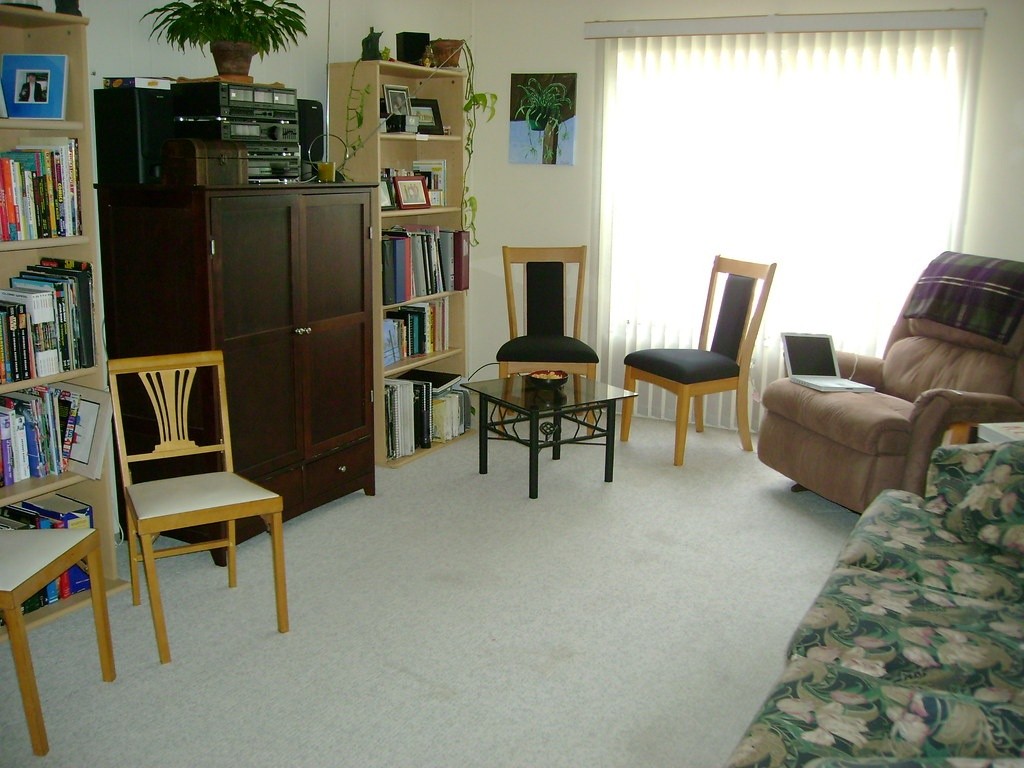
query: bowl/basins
[{"left": 529, "top": 370, "right": 568, "bottom": 389}]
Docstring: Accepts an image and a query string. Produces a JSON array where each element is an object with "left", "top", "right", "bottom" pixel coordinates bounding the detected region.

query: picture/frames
[
  {"left": 394, "top": 176, "right": 432, "bottom": 209},
  {"left": 411, "top": 98, "right": 444, "bottom": 135},
  {"left": 382, "top": 84, "right": 411, "bottom": 117},
  {"left": 56, "top": 382, "right": 114, "bottom": 480},
  {"left": 4, "top": 54, "right": 70, "bottom": 119},
  {"left": 379, "top": 177, "right": 396, "bottom": 210}
]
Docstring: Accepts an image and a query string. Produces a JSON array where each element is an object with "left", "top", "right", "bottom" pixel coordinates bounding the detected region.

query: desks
[{"left": 460, "top": 374, "right": 639, "bottom": 499}]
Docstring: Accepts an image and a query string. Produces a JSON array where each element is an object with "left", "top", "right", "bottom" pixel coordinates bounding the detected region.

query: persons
[
  {"left": 392, "top": 94, "right": 407, "bottom": 115},
  {"left": 18, "top": 74, "right": 44, "bottom": 103},
  {"left": 403, "top": 184, "right": 420, "bottom": 202}
]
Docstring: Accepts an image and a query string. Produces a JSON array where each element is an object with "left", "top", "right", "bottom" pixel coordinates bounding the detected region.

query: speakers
[
  {"left": 92, "top": 88, "right": 180, "bottom": 187},
  {"left": 297, "top": 99, "right": 324, "bottom": 164}
]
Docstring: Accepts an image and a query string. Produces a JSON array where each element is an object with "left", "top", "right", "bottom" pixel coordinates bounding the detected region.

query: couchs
[
  {"left": 760, "top": 251, "right": 1024, "bottom": 514},
  {"left": 731, "top": 442, "right": 1024, "bottom": 768}
]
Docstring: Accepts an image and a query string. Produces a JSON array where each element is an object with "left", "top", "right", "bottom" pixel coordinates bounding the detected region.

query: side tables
[{"left": 976, "top": 422, "right": 1024, "bottom": 443}]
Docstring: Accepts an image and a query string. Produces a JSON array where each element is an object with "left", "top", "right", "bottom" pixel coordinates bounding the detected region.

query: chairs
[
  {"left": 1, "top": 528, "right": 117, "bottom": 754},
  {"left": 498, "top": 246, "right": 599, "bottom": 441},
  {"left": 106, "top": 350, "right": 291, "bottom": 665},
  {"left": 620, "top": 254, "right": 778, "bottom": 469}
]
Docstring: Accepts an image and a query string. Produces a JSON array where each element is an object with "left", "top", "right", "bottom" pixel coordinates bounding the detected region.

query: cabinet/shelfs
[
  {"left": 145, "top": 182, "right": 377, "bottom": 562},
  {"left": 323, "top": 59, "right": 468, "bottom": 472},
  {"left": 0, "top": 8, "right": 132, "bottom": 636}
]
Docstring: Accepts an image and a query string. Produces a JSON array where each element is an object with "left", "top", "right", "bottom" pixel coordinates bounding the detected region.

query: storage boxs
[{"left": 160, "top": 138, "right": 249, "bottom": 184}]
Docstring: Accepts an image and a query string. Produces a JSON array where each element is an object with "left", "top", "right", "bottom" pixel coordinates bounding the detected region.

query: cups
[{"left": 318, "top": 162, "right": 335, "bottom": 182}]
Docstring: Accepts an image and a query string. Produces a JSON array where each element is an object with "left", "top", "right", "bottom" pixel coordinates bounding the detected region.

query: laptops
[{"left": 781, "top": 333, "right": 876, "bottom": 393}]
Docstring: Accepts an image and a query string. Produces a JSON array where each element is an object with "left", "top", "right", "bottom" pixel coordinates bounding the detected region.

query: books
[
  {"left": 382, "top": 224, "right": 470, "bottom": 304},
  {"left": 0, "top": 490, "right": 95, "bottom": 628},
  {"left": 385, "top": 370, "right": 472, "bottom": 459},
  {"left": 0, "top": 136, "right": 83, "bottom": 241},
  {"left": 381, "top": 168, "right": 407, "bottom": 206},
  {"left": 382, "top": 296, "right": 450, "bottom": 369},
  {"left": 0, "top": 53, "right": 70, "bottom": 121},
  {"left": 0, "top": 382, "right": 111, "bottom": 487},
  {"left": 0, "top": 256, "right": 98, "bottom": 384},
  {"left": 408, "top": 160, "right": 447, "bottom": 207}
]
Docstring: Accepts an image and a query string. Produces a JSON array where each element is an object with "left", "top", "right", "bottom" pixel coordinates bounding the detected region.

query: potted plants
[
  {"left": 434, "top": 39, "right": 498, "bottom": 248},
  {"left": 515, "top": 78, "right": 571, "bottom": 160},
  {"left": 141, "top": 0, "right": 307, "bottom": 76}
]
